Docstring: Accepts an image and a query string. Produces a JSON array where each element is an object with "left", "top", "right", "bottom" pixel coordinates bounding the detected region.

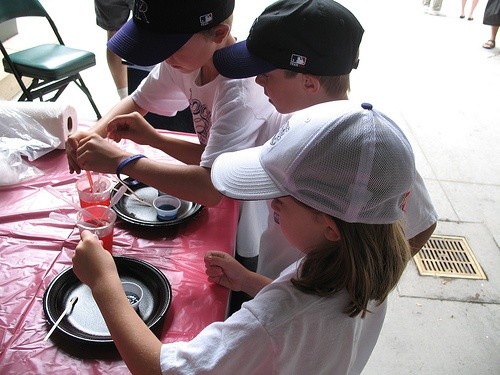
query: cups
[
  {"left": 74, "top": 205, "right": 117, "bottom": 255},
  {"left": 153, "top": 195, "right": 181, "bottom": 220},
  {"left": 121, "top": 282, "right": 143, "bottom": 311},
  {"left": 76, "top": 175, "right": 114, "bottom": 221}
]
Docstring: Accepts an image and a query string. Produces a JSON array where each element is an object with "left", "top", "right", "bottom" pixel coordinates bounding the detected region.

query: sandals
[{"left": 482, "top": 40, "right": 495, "bottom": 49}]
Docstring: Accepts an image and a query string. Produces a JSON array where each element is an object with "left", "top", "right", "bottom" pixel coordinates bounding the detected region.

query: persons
[
  {"left": 71, "top": 101, "right": 411, "bottom": 375},
  {"left": 423, "top": 0, "right": 500, "bottom": 101},
  {"left": 64, "top": 0, "right": 287, "bottom": 320},
  {"left": 106, "top": 0, "right": 438, "bottom": 280}
]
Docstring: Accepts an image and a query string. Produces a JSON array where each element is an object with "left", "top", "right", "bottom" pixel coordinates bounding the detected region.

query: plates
[
  {"left": 44, "top": 257, "right": 172, "bottom": 344},
  {"left": 111, "top": 177, "right": 204, "bottom": 228}
]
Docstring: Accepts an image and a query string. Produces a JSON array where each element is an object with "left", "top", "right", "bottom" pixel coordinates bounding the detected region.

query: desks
[{"left": 0, "top": 122, "right": 243, "bottom": 375}]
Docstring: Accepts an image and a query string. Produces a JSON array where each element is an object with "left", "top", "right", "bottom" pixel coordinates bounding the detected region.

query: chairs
[{"left": 0, "top": 0, "right": 102, "bottom": 121}]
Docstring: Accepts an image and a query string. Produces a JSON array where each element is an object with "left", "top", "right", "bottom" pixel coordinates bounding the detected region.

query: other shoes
[
  {"left": 460, "top": 16, "right": 465, "bottom": 18},
  {"left": 425, "top": 10, "right": 441, "bottom": 16},
  {"left": 468, "top": 18, "right": 473, "bottom": 20}
]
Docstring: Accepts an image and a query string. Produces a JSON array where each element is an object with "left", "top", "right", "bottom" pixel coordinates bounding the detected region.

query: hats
[
  {"left": 106, "top": 0, "right": 235, "bottom": 66},
  {"left": 211, "top": 100, "right": 415, "bottom": 224},
  {"left": 213, "top": 0, "right": 364, "bottom": 79}
]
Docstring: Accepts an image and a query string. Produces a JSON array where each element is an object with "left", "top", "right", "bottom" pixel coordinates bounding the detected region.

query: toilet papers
[{"left": 0, "top": 101, "right": 76, "bottom": 150}]
